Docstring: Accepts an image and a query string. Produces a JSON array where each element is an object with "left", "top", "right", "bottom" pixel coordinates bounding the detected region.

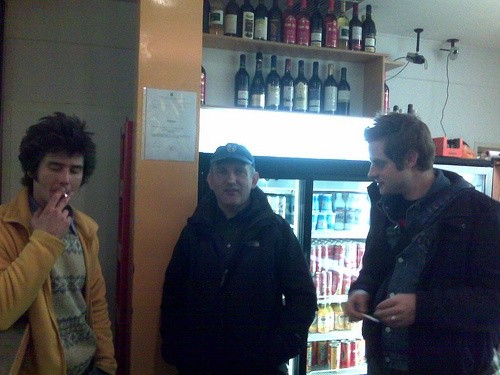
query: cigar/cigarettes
[
  {"left": 359, "top": 312, "right": 380, "bottom": 323},
  {"left": 64, "top": 193, "right": 68, "bottom": 198}
]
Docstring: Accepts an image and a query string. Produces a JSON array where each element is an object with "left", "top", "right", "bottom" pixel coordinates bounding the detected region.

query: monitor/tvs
[{"left": 434, "top": 165, "right": 492, "bottom": 198}]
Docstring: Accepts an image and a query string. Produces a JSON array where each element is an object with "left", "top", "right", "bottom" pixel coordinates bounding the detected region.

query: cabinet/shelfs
[{"left": 203, "top": 32, "right": 404, "bottom": 118}]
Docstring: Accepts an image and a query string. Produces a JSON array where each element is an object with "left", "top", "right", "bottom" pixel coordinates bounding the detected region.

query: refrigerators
[{"left": 198, "top": 151, "right": 373, "bottom": 375}]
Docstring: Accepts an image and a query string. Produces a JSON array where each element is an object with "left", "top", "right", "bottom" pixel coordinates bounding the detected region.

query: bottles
[
  {"left": 384, "top": 70, "right": 414, "bottom": 115},
  {"left": 234, "top": 54, "right": 250, "bottom": 108},
  {"left": 349, "top": 3, "right": 363, "bottom": 51},
  {"left": 336, "top": 0, "right": 349, "bottom": 50},
  {"left": 240, "top": 0, "right": 255, "bottom": 39},
  {"left": 225, "top": 0, "right": 240, "bottom": 38},
  {"left": 296, "top": 0, "right": 310, "bottom": 46},
  {"left": 280, "top": 58, "right": 294, "bottom": 112},
  {"left": 282, "top": 0, "right": 297, "bottom": 44},
  {"left": 334, "top": 193, "right": 371, "bottom": 230},
  {"left": 363, "top": 4, "right": 377, "bottom": 53},
  {"left": 294, "top": 60, "right": 308, "bottom": 113},
  {"left": 265, "top": 54, "right": 281, "bottom": 111},
  {"left": 337, "top": 67, "right": 351, "bottom": 116},
  {"left": 323, "top": 64, "right": 338, "bottom": 115},
  {"left": 308, "top": 61, "right": 323, "bottom": 114},
  {"left": 269, "top": 0, "right": 283, "bottom": 42},
  {"left": 309, "top": 0, "right": 324, "bottom": 47},
  {"left": 323, "top": 0, "right": 337, "bottom": 48},
  {"left": 255, "top": 0, "right": 268, "bottom": 40},
  {"left": 251, "top": 51, "right": 266, "bottom": 109},
  {"left": 200, "top": 65, "right": 206, "bottom": 108},
  {"left": 203, "top": 0, "right": 225, "bottom": 36}
]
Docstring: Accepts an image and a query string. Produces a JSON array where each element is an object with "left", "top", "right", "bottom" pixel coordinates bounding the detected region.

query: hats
[{"left": 210, "top": 143, "right": 255, "bottom": 168}]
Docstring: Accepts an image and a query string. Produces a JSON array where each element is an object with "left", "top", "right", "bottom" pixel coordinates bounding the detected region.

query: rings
[{"left": 392, "top": 315, "right": 396, "bottom": 324}]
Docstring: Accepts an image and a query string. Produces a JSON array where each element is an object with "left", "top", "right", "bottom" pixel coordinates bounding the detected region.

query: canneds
[
  {"left": 307, "top": 338, "right": 365, "bottom": 371},
  {"left": 310, "top": 242, "right": 365, "bottom": 296},
  {"left": 266, "top": 194, "right": 286, "bottom": 220}
]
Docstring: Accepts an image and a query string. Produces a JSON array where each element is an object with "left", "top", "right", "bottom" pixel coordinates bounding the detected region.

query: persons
[
  {"left": 153, "top": 142, "right": 319, "bottom": 375},
  {"left": 0, "top": 113, "right": 118, "bottom": 375},
  {"left": 343, "top": 112, "right": 500, "bottom": 375}
]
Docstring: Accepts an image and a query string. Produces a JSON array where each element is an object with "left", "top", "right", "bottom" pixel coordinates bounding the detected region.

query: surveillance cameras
[{"left": 406, "top": 52, "right": 426, "bottom": 64}]
